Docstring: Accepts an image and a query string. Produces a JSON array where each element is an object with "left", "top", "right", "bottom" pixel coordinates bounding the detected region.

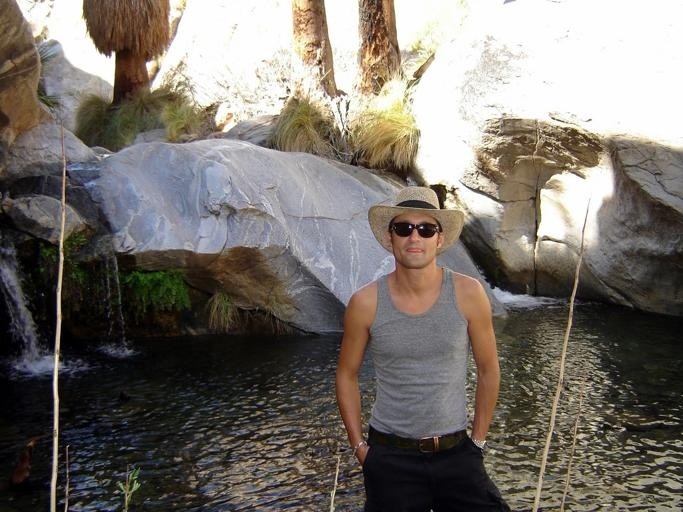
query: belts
[{"left": 368, "top": 424, "right": 467, "bottom": 455}]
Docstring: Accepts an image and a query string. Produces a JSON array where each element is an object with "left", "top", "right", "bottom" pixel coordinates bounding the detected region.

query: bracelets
[
  {"left": 351, "top": 439, "right": 366, "bottom": 460},
  {"left": 469, "top": 436, "right": 486, "bottom": 450}
]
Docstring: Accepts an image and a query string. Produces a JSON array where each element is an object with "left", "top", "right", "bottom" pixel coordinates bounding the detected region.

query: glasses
[{"left": 388, "top": 222, "right": 440, "bottom": 238}]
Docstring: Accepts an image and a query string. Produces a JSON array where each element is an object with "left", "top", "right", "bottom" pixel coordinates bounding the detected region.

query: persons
[{"left": 332, "top": 184, "right": 512, "bottom": 511}]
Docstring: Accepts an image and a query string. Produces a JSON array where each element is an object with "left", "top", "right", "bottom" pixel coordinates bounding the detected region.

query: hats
[{"left": 368, "top": 186, "right": 464, "bottom": 256}]
